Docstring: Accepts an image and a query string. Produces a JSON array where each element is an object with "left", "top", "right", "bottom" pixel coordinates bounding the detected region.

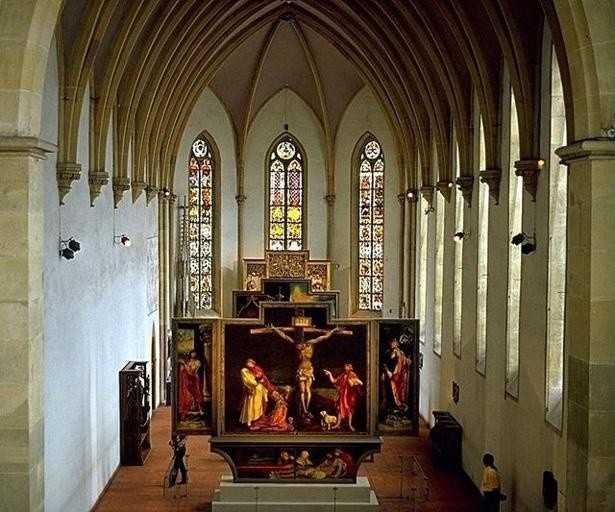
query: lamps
[
  {"left": 452, "top": 231, "right": 468, "bottom": 242},
  {"left": 114, "top": 234, "right": 131, "bottom": 247},
  {"left": 59, "top": 235, "right": 80, "bottom": 261},
  {"left": 511, "top": 231, "right": 536, "bottom": 255}
]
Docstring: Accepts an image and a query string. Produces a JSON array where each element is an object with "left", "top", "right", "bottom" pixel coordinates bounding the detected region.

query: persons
[
  {"left": 381, "top": 335, "right": 412, "bottom": 414},
  {"left": 250, "top": 367, "right": 294, "bottom": 433},
  {"left": 323, "top": 362, "right": 363, "bottom": 433},
  {"left": 177, "top": 348, "right": 206, "bottom": 424},
  {"left": 478, "top": 451, "right": 508, "bottom": 511},
  {"left": 269, "top": 448, "right": 352, "bottom": 480},
  {"left": 239, "top": 357, "right": 268, "bottom": 427},
  {"left": 264, "top": 320, "right": 346, "bottom": 419},
  {"left": 390, "top": 346, "right": 411, "bottom": 411},
  {"left": 167, "top": 431, "right": 188, "bottom": 488}
]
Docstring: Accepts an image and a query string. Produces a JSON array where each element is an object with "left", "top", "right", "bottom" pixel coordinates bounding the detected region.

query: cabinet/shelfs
[{"left": 120, "top": 358, "right": 152, "bottom": 467}]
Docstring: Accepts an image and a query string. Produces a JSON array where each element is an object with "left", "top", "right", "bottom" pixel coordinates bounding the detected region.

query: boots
[
  {"left": 168, "top": 475, "right": 176, "bottom": 487},
  {"left": 177, "top": 473, "right": 187, "bottom": 484}
]
